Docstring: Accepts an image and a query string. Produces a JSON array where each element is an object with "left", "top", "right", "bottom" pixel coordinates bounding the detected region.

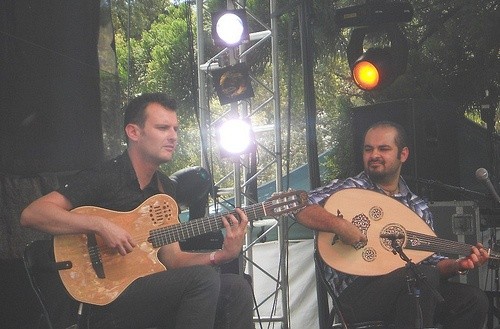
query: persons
[
  {"left": 285, "top": 120, "right": 491, "bottom": 329},
  {"left": 19, "top": 93, "right": 255, "bottom": 329}
]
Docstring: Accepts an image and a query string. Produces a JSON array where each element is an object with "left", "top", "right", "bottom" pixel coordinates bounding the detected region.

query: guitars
[
  {"left": 53, "top": 189, "right": 310, "bottom": 307},
  {"left": 316, "top": 188, "right": 500, "bottom": 277}
]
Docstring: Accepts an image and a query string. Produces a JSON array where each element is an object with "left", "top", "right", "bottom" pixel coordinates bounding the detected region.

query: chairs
[
  {"left": 314, "top": 229, "right": 445, "bottom": 329},
  {"left": 21, "top": 235, "right": 77, "bottom": 329}
]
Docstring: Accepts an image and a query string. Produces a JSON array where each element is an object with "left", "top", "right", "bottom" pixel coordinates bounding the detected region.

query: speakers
[{"left": 350, "top": 97, "right": 418, "bottom": 195}]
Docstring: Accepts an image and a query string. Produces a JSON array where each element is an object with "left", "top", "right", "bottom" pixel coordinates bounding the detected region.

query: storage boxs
[{"left": 426, "top": 201, "right": 484, "bottom": 291}]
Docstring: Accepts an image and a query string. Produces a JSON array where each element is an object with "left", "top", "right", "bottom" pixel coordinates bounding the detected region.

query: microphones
[{"left": 475, "top": 167, "right": 500, "bottom": 205}]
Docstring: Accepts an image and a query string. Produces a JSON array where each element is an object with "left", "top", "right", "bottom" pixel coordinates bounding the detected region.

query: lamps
[
  {"left": 210, "top": 62, "right": 254, "bottom": 106},
  {"left": 334, "top": 1, "right": 414, "bottom": 93}
]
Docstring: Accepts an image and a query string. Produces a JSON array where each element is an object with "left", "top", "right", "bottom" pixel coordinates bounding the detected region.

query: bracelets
[
  {"left": 209, "top": 248, "right": 222, "bottom": 265},
  {"left": 455, "top": 256, "right": 470, "bottom": 275}
]
